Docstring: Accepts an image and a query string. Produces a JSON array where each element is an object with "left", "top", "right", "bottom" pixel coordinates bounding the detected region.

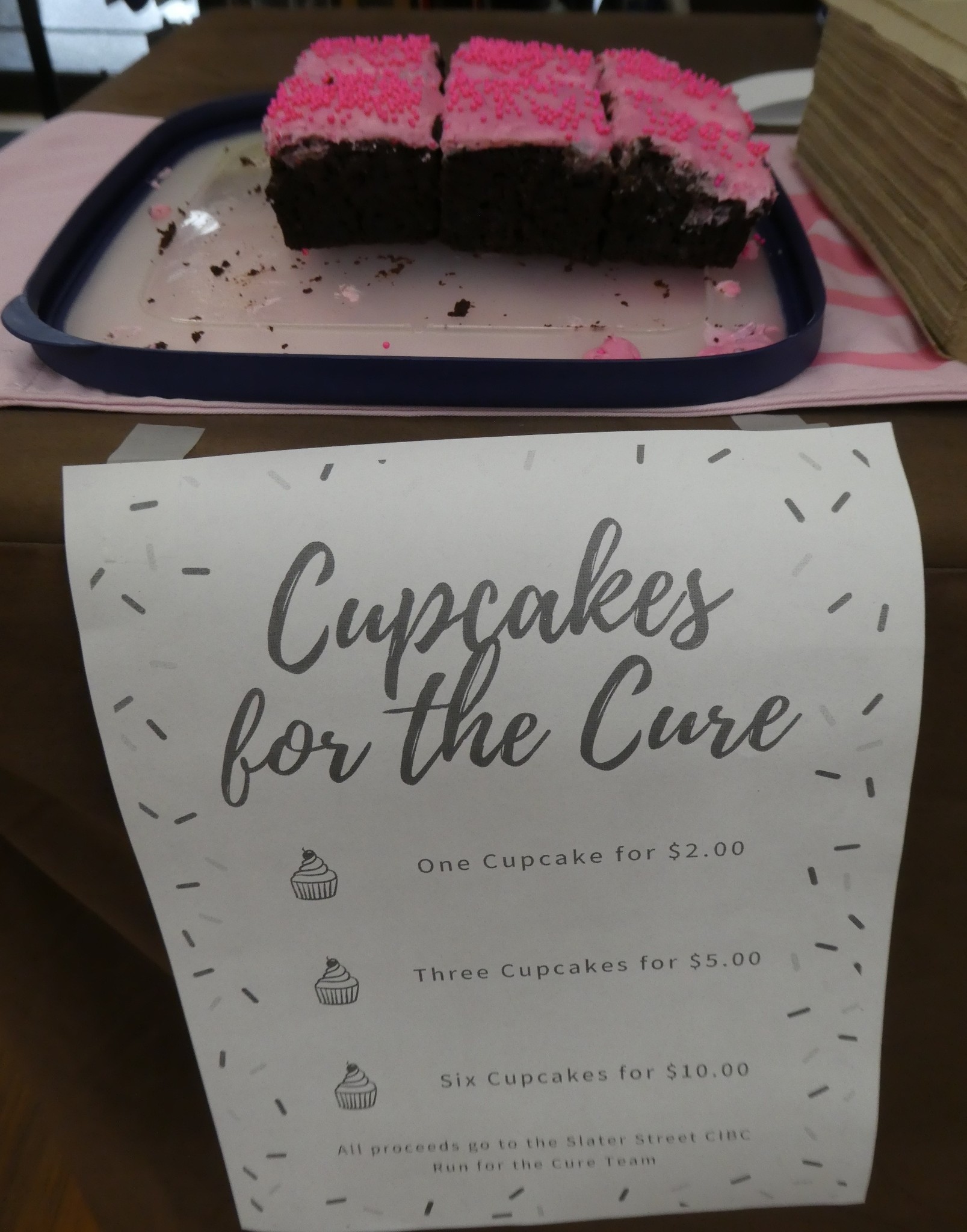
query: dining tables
[{"left": 0, "top": 2, "right": 967, "bottom": 1232}]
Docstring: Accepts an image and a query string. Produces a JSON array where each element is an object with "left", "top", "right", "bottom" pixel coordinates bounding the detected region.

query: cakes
[{"left": 265, "top": 37, "right": 775, "bottom": 269}]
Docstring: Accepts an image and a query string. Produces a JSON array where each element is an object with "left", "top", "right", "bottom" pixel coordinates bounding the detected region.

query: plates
[{"left": 3, "top": 89, "right": 825, "bottom": 408}]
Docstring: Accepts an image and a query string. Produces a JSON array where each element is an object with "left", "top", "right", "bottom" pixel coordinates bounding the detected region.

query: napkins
[{"left": 793, "top": 1, "right": 967, "bottom": 369}]
